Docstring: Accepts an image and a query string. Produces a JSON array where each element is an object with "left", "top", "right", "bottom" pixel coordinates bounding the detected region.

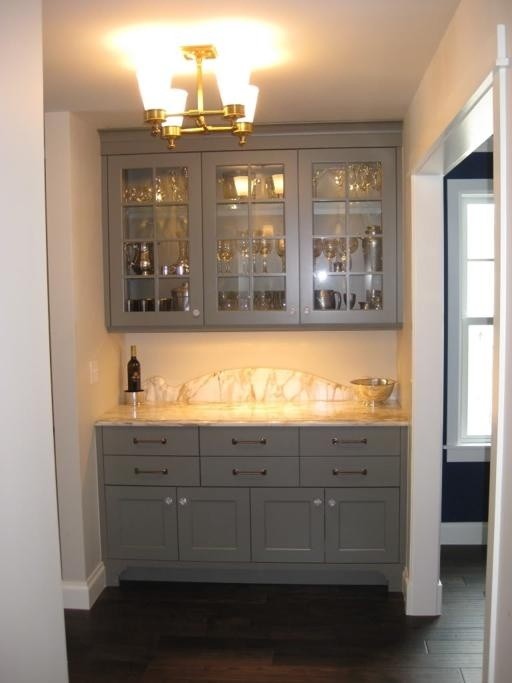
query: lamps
[{"left": 135, "top": 44, "right": 259, "bottom": 151}]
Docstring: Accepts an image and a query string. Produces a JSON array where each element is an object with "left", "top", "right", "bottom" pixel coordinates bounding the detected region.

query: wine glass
[{"left": 217, "top": 236, "right": 360, "bottom": 274}]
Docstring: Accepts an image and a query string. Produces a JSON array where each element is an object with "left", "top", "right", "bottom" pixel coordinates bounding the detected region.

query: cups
[
  {"left": 344, "top": 293, "right": 355, "bottom": 309},
  {"left": 314, "top": 290, "right": 342, "bottom": 311}
]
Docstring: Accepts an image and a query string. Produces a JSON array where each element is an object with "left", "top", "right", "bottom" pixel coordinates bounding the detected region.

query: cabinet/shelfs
[
  {"left": 299, "top": 427, "right": 401, "bottom": 487},
  {"left": 98, "top": 120, "right": 404, "bottom": 330},
  {"left": 99, "top": 427, "right": 201, "bottom": 488},
  {"left": 200, "top": 426, "right": 300, "bottom": 488},
  {"left": 102, "top": 485, "right": 251, "bottom": 563},
  {"left": 250, "top": 487, "right": 400, "bottom": 565}
]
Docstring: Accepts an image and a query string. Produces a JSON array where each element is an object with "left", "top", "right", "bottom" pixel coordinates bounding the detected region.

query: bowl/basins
[{"left": 350, "top": 377, "right": 396, "bottom": 401}]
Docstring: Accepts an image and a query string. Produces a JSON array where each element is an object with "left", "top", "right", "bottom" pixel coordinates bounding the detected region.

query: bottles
[
  {"left": 127, "top": 344, "right": 140, "bottom": 392},
  {"left": 127, "top": 239, "right": 188, "bottom": 313},
  {"left": 362, "top": 223, "right": 383, "bottom": 274}
]
